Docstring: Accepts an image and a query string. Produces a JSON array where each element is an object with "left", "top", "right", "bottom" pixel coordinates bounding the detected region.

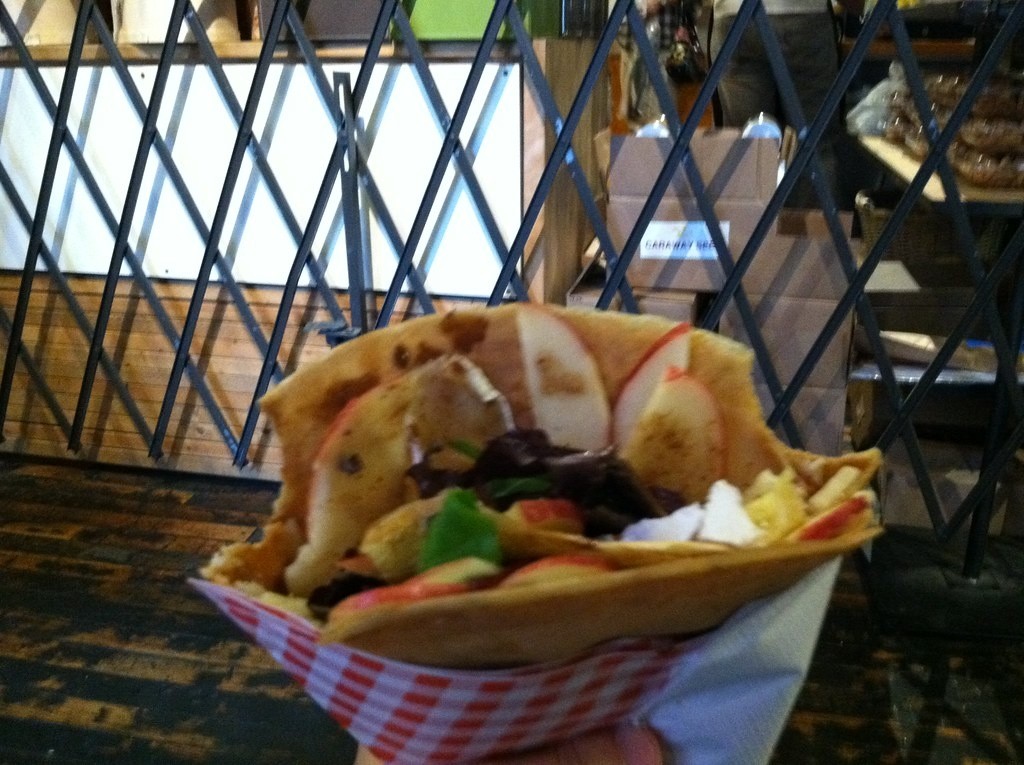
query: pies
[{"left": 192, "top": 303, "right": 885, "bottom": 668}]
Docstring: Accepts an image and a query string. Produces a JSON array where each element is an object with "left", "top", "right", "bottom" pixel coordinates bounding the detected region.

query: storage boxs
[
  {"left": 0, "top": 0, "right": 607, "bottom": 46},
  {"left": 567, "top": 129, "right": 920, "bottom": 457}
]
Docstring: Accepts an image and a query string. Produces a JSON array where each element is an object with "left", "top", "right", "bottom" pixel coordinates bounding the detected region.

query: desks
[{"left": 858, "top": 134, "right": 1024, "bottom": 639}]
